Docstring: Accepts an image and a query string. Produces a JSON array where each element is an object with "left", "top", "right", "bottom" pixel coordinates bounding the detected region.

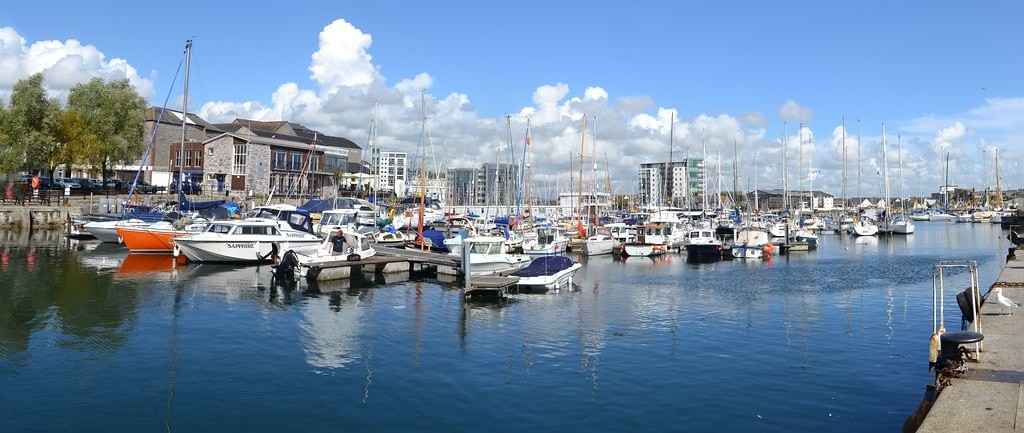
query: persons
[{"left": 327, "top": 229, "right": 351, "bottom": 256}]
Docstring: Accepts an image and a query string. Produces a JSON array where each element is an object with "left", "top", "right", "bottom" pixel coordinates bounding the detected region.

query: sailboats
[{"left": 61, "top": 38, "right": 1024, "bottom": 311}]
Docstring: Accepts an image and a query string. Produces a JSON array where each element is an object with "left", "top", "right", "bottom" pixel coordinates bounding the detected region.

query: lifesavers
[{"left": 32, "top": 176, "right": 40, "bottom": 188}]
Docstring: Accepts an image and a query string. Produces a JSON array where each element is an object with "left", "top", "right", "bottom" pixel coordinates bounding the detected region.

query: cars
[{"left": 10, "top": 175, "right": 202, "bottom": 198}]
